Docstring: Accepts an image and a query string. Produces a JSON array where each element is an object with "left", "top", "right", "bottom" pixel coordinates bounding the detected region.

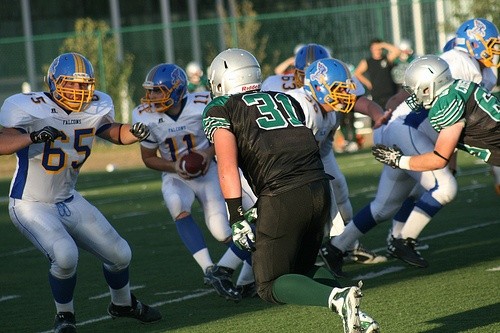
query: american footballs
[{"left": 180, "top": 152, "right": 206, "bottom": 177}]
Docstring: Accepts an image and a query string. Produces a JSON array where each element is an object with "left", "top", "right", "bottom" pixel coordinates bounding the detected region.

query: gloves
[
  {"left": 244, "top": 207, "right": 258, "bottom": 224},
  {"left": 225, "top": 220, "right": 255, "bottom": 251},
  {"left": 30, "top": 125, "right": 61, "bottom": 144},
  {"left": 373, "top": 144, "right": 401, "bottom": 168},
  {"left": 129, "top": 122, "right": 149, "bottom": 139}
]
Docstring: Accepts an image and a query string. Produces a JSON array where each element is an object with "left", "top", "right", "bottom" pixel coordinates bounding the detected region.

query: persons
[
  {"left": 131, "top": 64, "right": 234, "bottom": 275},
  {"left": 261, "top": 18, "right": 500, "bottom": 280},
  {"left": 202, "top": 57, "right": 358, "bottom": 303},
  {"left": 1, "top": 53, "right": 163, "bottom": 333},
  {"left": 201, "top": 49, "right": 380, "bottom": 333},
  {"left": 184, "top": 61, "right": 211, "bottom": 94}
]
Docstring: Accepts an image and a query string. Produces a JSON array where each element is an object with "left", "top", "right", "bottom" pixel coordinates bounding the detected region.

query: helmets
[
  {"left": 141, "top": 64, "right": 189, "bottom": 116},
  {"left": 46, "top": 51, "right": 97, "bottom": 112},
  {"left": 305, "top": 58, "right": 356, "bottom": 115},
  {"left": 456, "top": 19, "right": 500, "bottom": 68},
  {"left": 293, "top": 44, "right": 330, "bottom": 87},
  {"left": 207, "top": 48, "right": 262, "bottom": 98},
  {"left": 406, "top": 55, "right": 454, "bottom": 105}
]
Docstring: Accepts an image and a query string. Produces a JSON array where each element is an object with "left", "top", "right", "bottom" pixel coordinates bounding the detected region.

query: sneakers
[
  {"left": 388, "top": 230, "right": 429, "bottom": 252},
  {"left": 341, "top": 245, "right": 377, "bottom": 264},
  {"left": 106, "top": 291, "right": 161, "bottom": 325},
  {"left": 386, "top": 238, "right": 427, "bottom": 269},
  {"left": 359, "top": 311, "right": 380, "bottom": 333},
  {"left": 320, "top": 236, "right": 346, "bottom": 279},
  {"left": 232, "top": 280, "right": 256, "bottom": 299},
  {"left": 327, "top": 286, "right": 360, "bottom": 333},
  {"left": 53, "top": 311, "right": 78, "bottom": 332},
  {"left": 208, "top": 265, "right": 242, "bottom": 301}
]
